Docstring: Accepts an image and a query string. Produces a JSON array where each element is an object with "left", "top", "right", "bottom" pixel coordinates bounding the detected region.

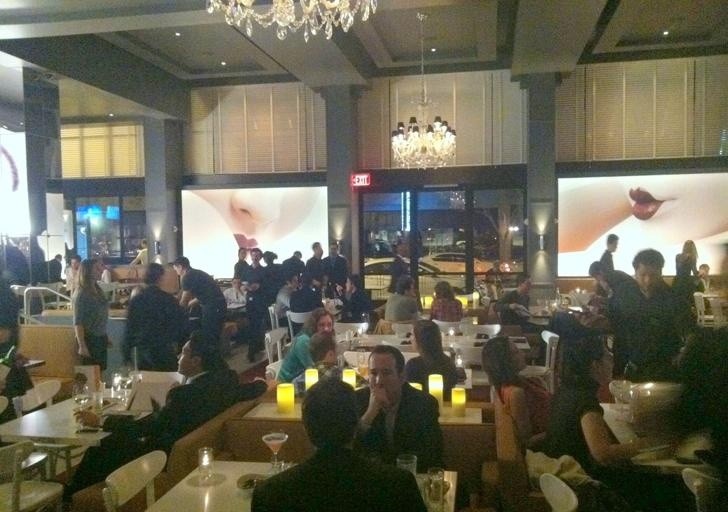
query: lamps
[
  {"left": 391, "top": 13, "right": 456, "bottom": 171},
  {"left": 206, "top": 1, "right": 377, "bottom": 41}
]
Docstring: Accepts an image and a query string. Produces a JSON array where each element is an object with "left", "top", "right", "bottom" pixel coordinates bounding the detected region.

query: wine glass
[
  {"left": 612, "top": 377, "right": 631, "bottom": 422},
  {"left": 67, "top": 366, "right": 132, "bottom": 428},
  {"left": 261, "top": 432, "right": 289, "bottom": 474}
]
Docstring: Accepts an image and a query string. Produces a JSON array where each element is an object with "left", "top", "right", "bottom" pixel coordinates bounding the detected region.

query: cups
[
  {"left": 428, "top": 374, "right": 443, "bottom": 407},
  {"left": 450, "top": 388, "right": 466, "bottom": 417},
  {"left": 304, "top": 369, "right": 319, "bottom": 392},
  {"left": 132, "top": 371, "right": 143, "bottom": 385},
  {"left": 341, "top": 369, "right": 356, "bottom": 390},
  {"left": 275, "top": 383, "right": 295, "bottom": 415},
  {"left": 408, "top": 382, "right": 422, "bottom": 390},
  {"left": 425, "top": 468, "right": 445, "bottom": 512},
  {"left": 197, "top": 447, "right": 215, "bottom": 481},
  {"left": 356, "top": 347, "right": 369, "bottom": 377},
  {"left": 396, "top": 453, "right": 418, "bottom": 477},
  {"left": 345, "top": 329, "right": 354, "bottom": 346},
  {"left": 447, "top": 328, "right": 456, "bottom": 345},
  {"left": 535, "top": 286, "right": 562, "bottom": 315}
]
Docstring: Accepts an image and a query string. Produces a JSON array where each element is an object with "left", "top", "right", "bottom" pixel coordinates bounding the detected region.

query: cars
[
  {"left": 421, "top": 250, "right": 496, "bottom": 278},
  {"left": 358, "top": 260, "right": 478, "bottom": 302}
]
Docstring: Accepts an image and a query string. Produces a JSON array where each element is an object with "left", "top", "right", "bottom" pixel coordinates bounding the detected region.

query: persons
[
  {"left": 481, "top": 234, "right": 728, "bottom": 511},
  {"left": 1, "top": 239, "right": 463, "bottom": 511},
  {"left": 181, "top": 186, "right": 322, "bottom": 279},
  {"left": 558, "top": 173, "right": 728, "bottom": 274}
]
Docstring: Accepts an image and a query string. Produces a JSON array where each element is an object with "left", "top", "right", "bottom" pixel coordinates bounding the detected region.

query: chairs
[{"left": 0, "top": 293, "right": 728, "bottom": 512}]
